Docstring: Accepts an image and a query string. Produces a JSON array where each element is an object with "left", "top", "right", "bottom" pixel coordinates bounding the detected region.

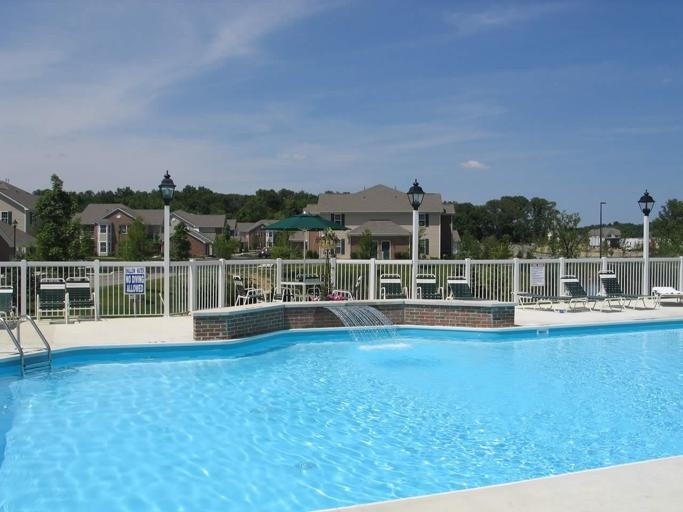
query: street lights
[
  {"left": 637, "top": 189, "right": 655, "bottom": 295},
  {"left": 157, "top": 169, "right": 176, "bottom": 318},
  {"left": 406, "top": 178, "right": 426, "bottom": 300}
]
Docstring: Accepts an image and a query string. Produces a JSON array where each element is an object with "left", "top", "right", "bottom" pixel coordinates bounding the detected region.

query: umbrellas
[{"left": 260, "top": 216, "right": 355, "bottom": 300}]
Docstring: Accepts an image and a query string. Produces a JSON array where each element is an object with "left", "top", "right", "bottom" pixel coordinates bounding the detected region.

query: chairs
[
  {"left": 379, "top": 273, "right": 479, "bottom": 301},
  {"left": 0, "top": 276, "right": 98, "bottom": 327},
  {"left": 233, "top": 272, "right": 355, "bottom": 306},
  {"left": 511, "top": 270, "right": 683, "bottom": 312}
]
